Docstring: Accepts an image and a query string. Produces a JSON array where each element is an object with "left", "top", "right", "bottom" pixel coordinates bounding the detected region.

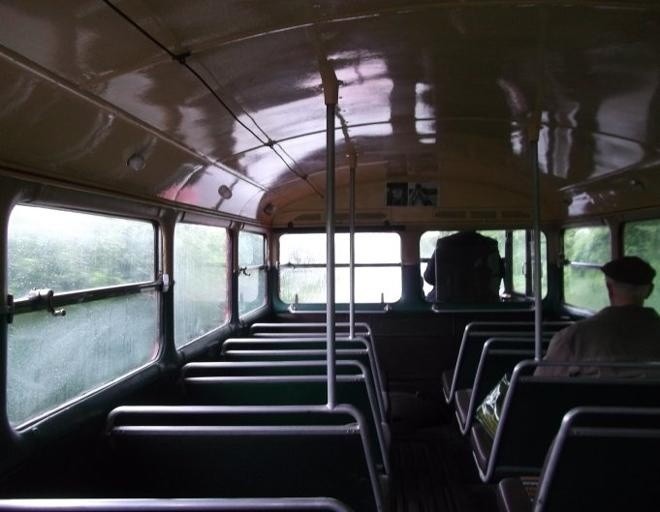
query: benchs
[
  {"left": 440, "top": 317, "right": 660, "bottom": 511},
  {"left": 1, "top": 320, "right": 397, "bottom": 511}
]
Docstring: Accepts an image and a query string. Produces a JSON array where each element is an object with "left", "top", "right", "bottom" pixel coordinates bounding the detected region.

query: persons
[
  {"left": 422, "top": 229, "right": 505, "bottom": 304},
  {"left": 534, "top": 255, "right": 660, "bottom": 383}
]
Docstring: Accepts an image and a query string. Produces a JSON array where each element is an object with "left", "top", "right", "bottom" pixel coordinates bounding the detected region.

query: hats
[{"left": 599, "top": 256, "right": 657, "bottom": 284}]
